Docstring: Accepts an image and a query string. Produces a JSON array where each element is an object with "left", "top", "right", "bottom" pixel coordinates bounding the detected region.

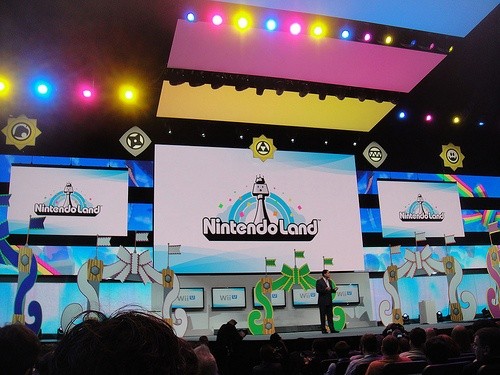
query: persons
[
  {"left": 316, "top": 270, "right": 339, "bottom": 333},
  {"left": 0, "top": 311, "right": 500, "bottom": 375}
]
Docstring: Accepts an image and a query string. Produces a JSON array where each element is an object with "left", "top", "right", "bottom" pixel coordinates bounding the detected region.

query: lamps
[
  {"left": 436, "top": 311, "right": 442, "bottom": 320},
  {"left": 402, "top": 313, "right": 409, "bottom": 320},
  {"left": 57, "top": 327, "right": 65, "bottom": 337},
  {"left": 481, "top": 307, "right": 489, "bottom": 318}
]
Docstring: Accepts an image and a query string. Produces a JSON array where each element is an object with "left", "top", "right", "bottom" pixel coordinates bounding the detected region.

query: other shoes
[
  {"left": 331, "top": 330, "right": 339, "bottom": 333},
  {"left": 322, "top": 331, "right": 328, "bottom": 333}
]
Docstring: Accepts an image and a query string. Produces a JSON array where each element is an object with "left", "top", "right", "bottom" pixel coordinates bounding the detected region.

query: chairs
[{"left": 321, "top": 348, "right": 476, "bottom": 375}]
